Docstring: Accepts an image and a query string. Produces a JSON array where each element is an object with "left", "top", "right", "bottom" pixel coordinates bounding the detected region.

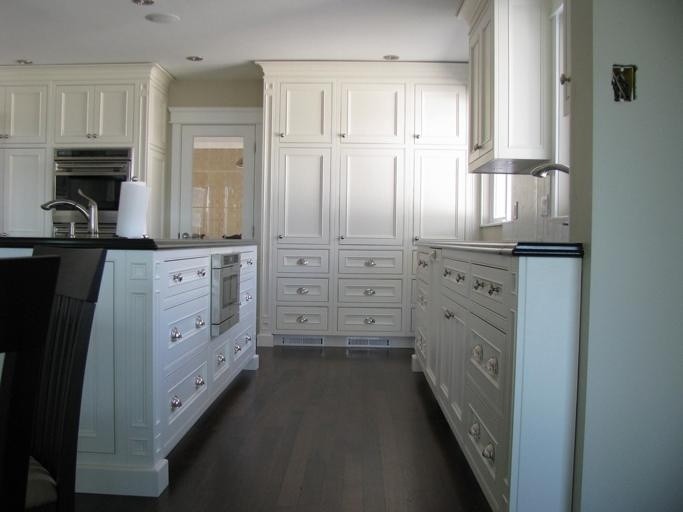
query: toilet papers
[{"left": 116, "top": 178, "right": 150, "bottom": 239}]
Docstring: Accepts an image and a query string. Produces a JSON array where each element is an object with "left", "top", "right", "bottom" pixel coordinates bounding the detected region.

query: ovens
[
  {"left": 50, "top": 146, "right": 135, "bottom": 239},
  {"left": 209, "top": 252, "right": 243, "bottom": 339}
]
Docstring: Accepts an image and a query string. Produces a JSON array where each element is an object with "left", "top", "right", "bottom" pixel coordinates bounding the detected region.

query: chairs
[{"left": 1, "top": 241, "right": 109, "bottom": 511}]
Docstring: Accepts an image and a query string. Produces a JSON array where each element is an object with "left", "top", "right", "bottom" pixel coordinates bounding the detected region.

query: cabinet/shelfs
[
  {"left": 116, "top": 247, "right": 261, "bottom": 499},
  {"left": 2, "top": 150, "right": 51, "bottom": 238},
  {"left": 465, "top": 249, "right": 583, "bottom": 512},
  {"left": 258, "top": 247, "right": 409, "bottom": 347},
  {"left": 269, "top": 77, "right": 470, "bottom": 245},
  {"left": 413, "top": 250, "right": 471, "bottom": 447},
  {"left": 467, "top": 0, "right": 558, "bottom": 176},
  {"left": 0, "top": 70, "right": 168, "bottom": 146}
]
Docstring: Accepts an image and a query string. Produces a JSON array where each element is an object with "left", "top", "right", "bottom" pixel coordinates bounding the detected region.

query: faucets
[
  {"left": 530, "top": 164, "right": 570, "bottom": 180},
  {"left": 41, "top": 188, "right": 99, "bottom": 233}
]
